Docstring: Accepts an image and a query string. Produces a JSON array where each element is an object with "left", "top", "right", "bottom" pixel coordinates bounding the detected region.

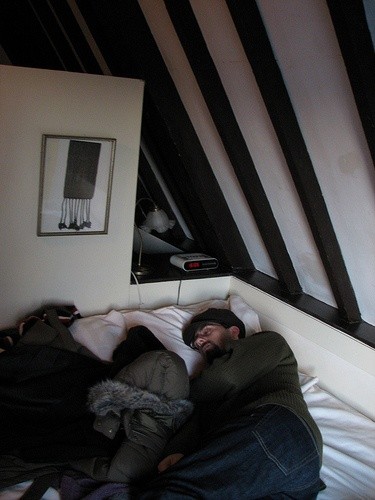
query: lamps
[{"left": 134, "top": 196, "right": 175, "bottom": 277}]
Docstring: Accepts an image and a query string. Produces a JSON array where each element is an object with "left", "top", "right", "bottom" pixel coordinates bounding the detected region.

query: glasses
[{"left": 190, "top": 324, "right": 223, "bottom": 348}]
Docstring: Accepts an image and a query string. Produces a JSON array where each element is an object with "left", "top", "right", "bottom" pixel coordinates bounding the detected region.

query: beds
[{"left": 1, "top": 292, "right": 375, "bottom": 500}]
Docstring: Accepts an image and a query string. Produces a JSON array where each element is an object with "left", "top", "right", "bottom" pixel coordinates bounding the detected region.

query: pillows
[{"left": 68, "top": 294, "right": 263, "bottom": 378}]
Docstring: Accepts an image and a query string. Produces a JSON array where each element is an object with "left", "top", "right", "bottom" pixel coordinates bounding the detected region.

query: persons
[{"left": 140, "top": 307, "right": 323, "bottom": 499}]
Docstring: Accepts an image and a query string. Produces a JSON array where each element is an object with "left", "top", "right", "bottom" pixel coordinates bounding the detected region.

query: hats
[{"left": 183, "top": 308, "right": 246, "bottom": 348}]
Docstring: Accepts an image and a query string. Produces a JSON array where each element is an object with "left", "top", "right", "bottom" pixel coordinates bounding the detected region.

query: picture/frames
[{"left": 36, "top": 133, "right": 118, "bottom": 237}]
